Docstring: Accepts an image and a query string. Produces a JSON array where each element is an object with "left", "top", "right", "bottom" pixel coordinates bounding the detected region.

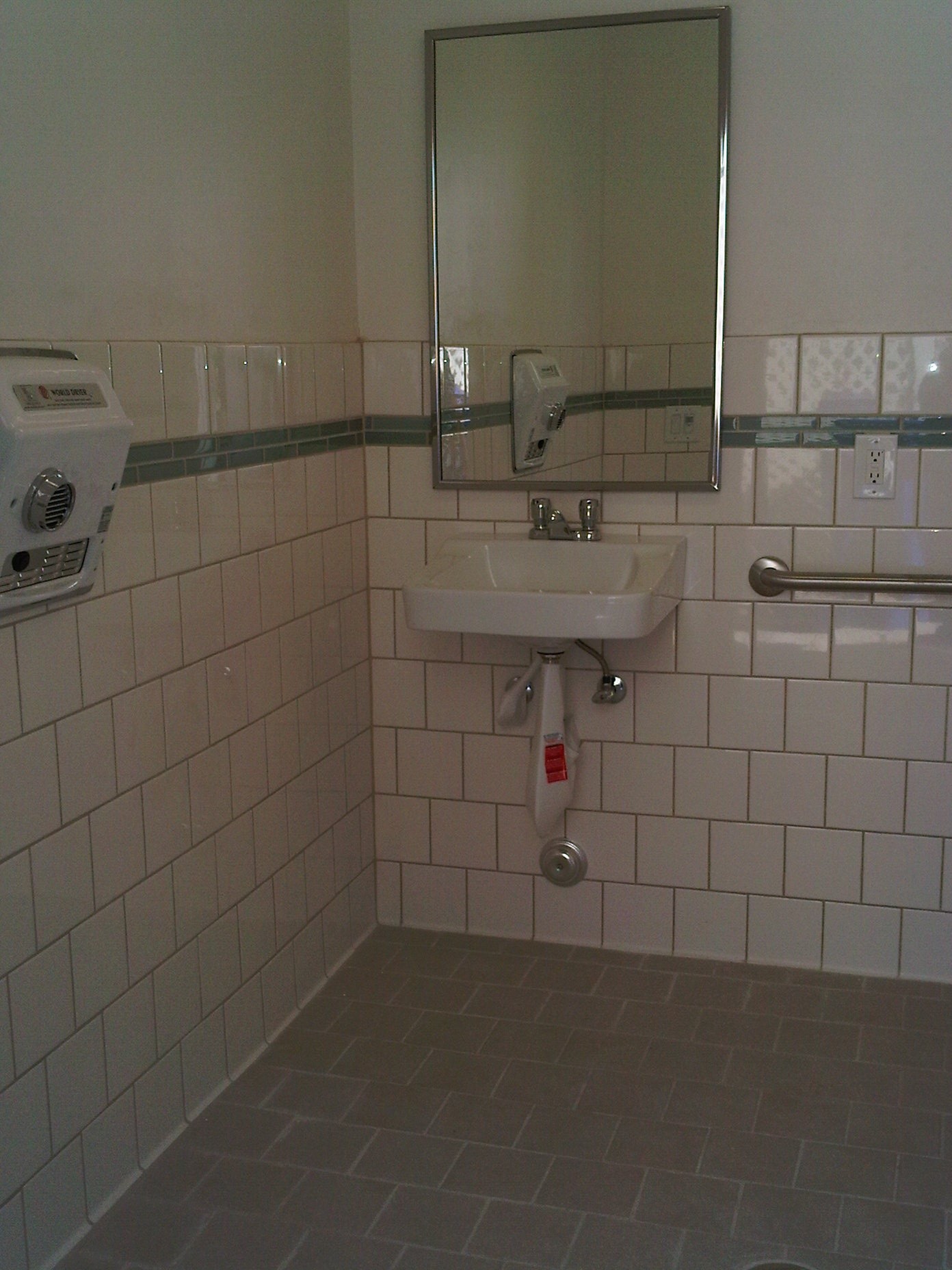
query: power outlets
[{"left": 853, "top": 433, "right": 899, "bottom": 498}]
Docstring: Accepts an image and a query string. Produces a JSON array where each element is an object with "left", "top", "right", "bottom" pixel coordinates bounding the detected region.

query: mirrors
[{"left": 424, "top": 6, "right": 731, "bottom": 491}]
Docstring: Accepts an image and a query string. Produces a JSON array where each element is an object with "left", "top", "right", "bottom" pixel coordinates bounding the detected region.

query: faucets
[{"left": 547, "top": 509, "right": 572, "bottom": 540}]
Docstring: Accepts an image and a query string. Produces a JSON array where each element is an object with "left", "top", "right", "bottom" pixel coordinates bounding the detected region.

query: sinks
[{"left": 403, "top": 532, "right": 689, "bottom": 641}]
